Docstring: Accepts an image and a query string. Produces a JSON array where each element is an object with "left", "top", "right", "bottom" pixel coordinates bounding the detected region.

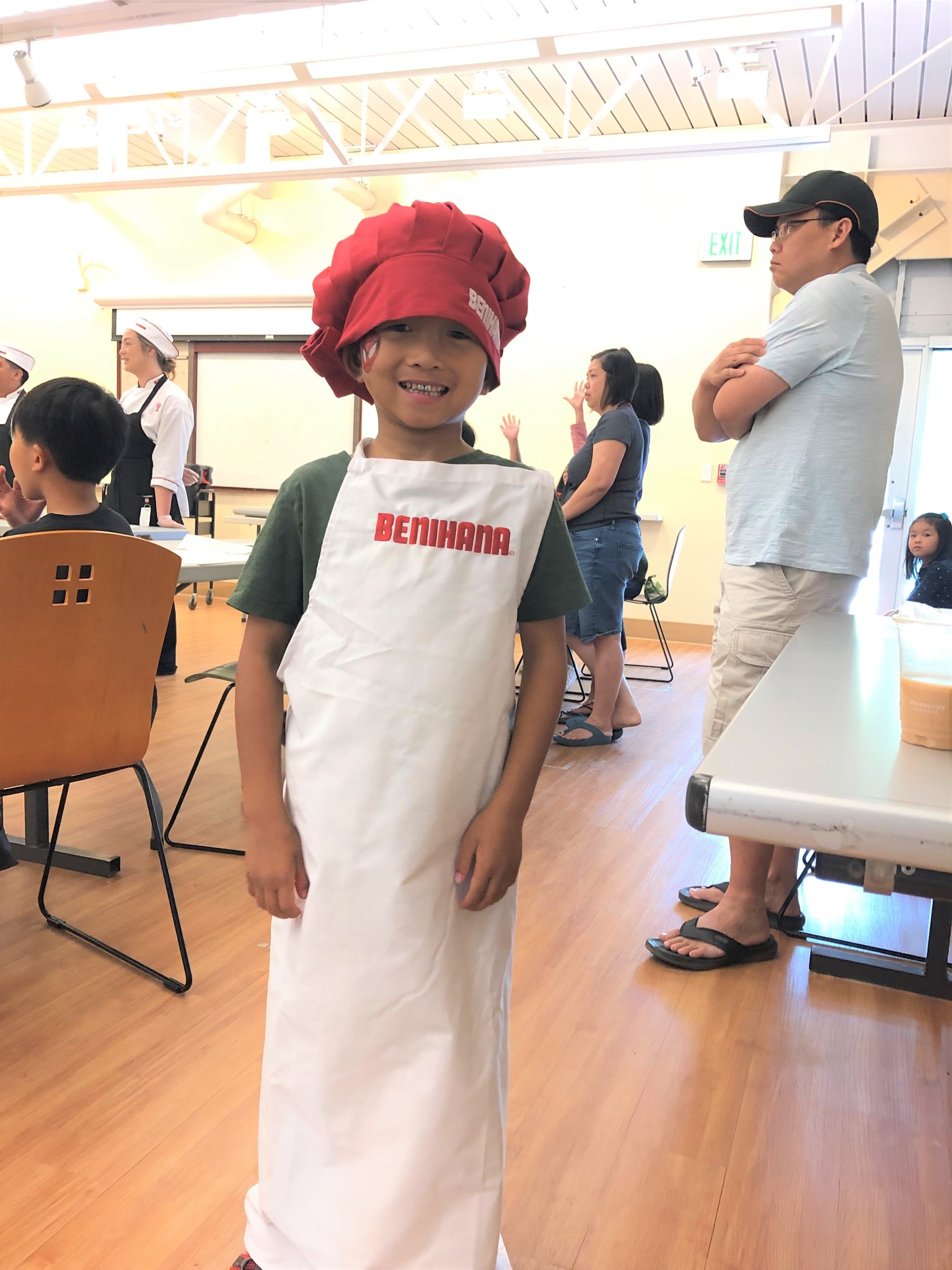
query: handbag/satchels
[{"left": 644, "top": 574, "right": 665, "bottom": 602}]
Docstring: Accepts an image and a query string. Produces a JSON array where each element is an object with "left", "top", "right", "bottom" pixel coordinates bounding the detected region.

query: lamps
[{"left": 13, "top": 37, "right": 54, "bottom": 108}]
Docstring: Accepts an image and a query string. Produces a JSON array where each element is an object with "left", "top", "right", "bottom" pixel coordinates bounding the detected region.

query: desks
[
  {"left": 686, "top": 609, "right": 952, "bottom": 1004},
  {"left": 1, "top": 524, "right": 254, "bottom": 878},
  {"left": 234, "top": 506, "right": 275, "bottom": 538}
]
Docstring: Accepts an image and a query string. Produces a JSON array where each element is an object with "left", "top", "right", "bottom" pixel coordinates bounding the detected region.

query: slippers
[
  {"left": 679, "top": 882, "right": 805, "bottom": 931},
  {"left": 553, "top": 723, "right": 612, "bottom": 747},
  {"left": 566, "top": 716, "right": 623, "bottom": 739},
  {"left": 646, "top": 916, "right": 777, "bottom": 969}
]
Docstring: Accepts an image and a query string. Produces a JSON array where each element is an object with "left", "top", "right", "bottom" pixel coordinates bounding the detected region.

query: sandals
[{"left": 558, "top": 704, "right": 593, "bottom": 723}]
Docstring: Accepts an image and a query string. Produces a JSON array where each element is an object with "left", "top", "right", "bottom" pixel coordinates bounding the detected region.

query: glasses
[{"left": 771, "top": 216, "right": 841, "bottom": 241}]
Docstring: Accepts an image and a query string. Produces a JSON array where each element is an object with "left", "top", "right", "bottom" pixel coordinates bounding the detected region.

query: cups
[{"left": 892, "top": 608, "right": 952, "bottom": 751}]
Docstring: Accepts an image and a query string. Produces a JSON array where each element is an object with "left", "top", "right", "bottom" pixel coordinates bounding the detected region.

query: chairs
[
  {"left": 0, "top": 530, "right": 192, "bottom": 993},
  {"left": 164, "top": 660, "right": 289, "bottom": 857},
  {"left": 582, "top": 522, "right": 688, "bottom": 682}
]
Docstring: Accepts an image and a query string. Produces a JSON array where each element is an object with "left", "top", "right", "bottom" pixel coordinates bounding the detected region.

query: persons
[
  {"left": 0, "top": 345, "right": 178, "bottom": 732},
  {"left": 500, "top": 346, "right": 665, "bottom": 747},
  {"left": 225, "top": 199, "right": 593, "bottom": 1270},
  {"left": 644, "top": 170, "right": 905, "bottom": 971},
  {"left": 904, "top": 512, "right": 952, "bottom": 609},
  {"left": 101, "top": 316, "right": 200, "bottom": 530}
]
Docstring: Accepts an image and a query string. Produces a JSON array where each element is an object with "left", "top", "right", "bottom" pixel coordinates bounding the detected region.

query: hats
[
  {"left": 126, "top": 317, "right": 178, "bottom": 358},
  {"left": 744, "top": 171, "right": 879, "bottom": 244},
  {"left": 0, "top": 345, "right": 35, "bottom": 373},
  {"left": 298, "top": 200, "right": 529, "bottom": 404}
]
